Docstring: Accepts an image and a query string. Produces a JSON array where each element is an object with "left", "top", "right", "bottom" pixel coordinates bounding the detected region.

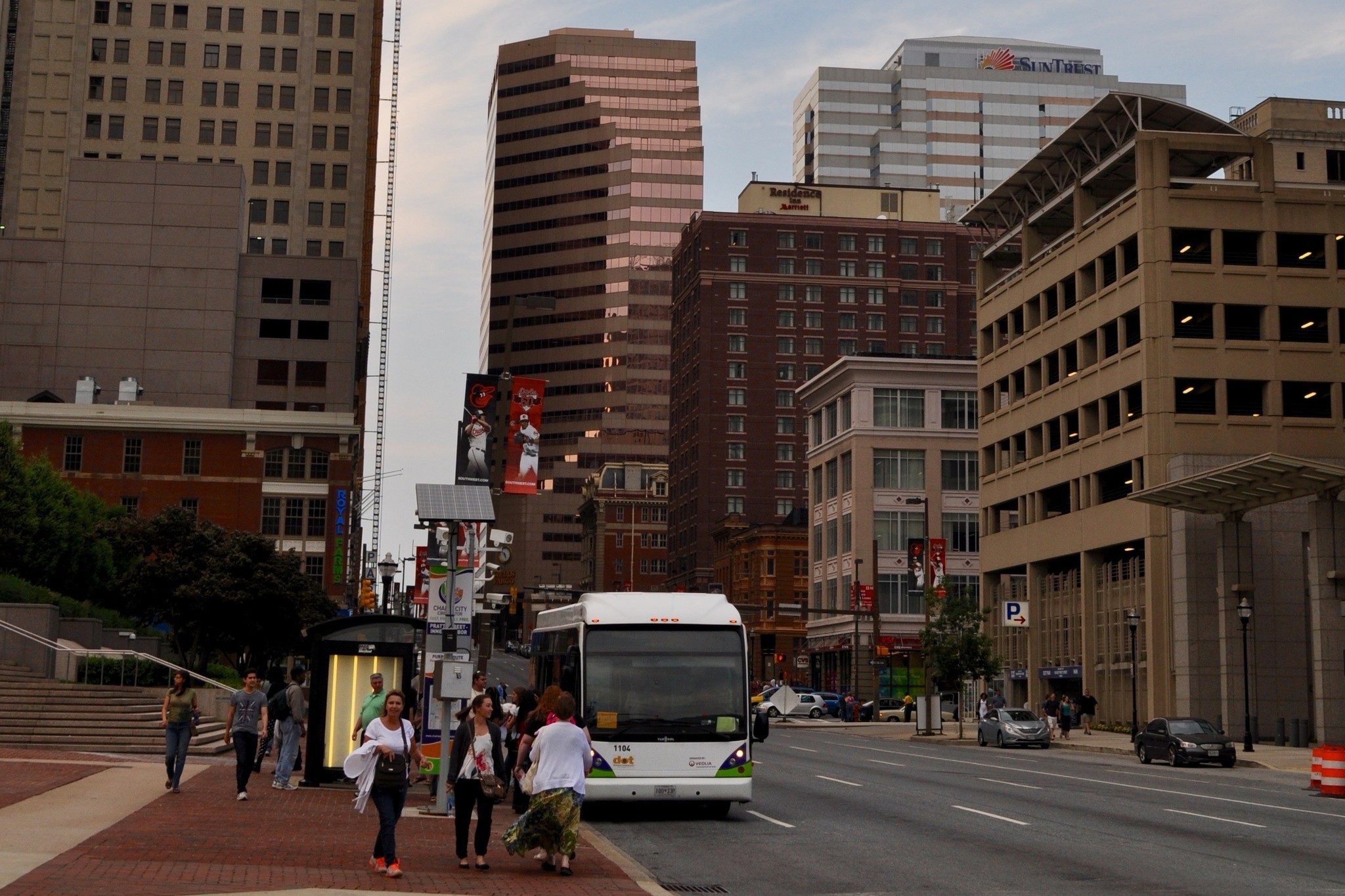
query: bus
[{"left": 530, "top": 592, "right": 769, "bottom": 816}]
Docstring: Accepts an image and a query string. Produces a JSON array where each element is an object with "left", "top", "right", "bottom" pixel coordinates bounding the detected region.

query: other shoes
[
  {"left": 1051, "top": 734, "right": 1055, "bottom": 740},
  {"left": 265, "top": 750, "right": 270, "bottom": 756},
  {"left": 475, "top": 863, "right": 490, "bottom": 870},
  {"left": 251, "top": 762, "right": 261, "bottom": 773},
  {"left": 840, "top": 719, "right": 854, "bottom": 722},
  {"left": 271, "top": 770, "right": 276, "bottom": 774},
  {"left": 540, "top": 861, "right": 557, "bottom": 872},
  {"left": 459, "top": 863, "right": 469, "bottom": 868},
  {"left": 1060, "top": 733, "right": 1070, "bottom": 739},
  {"left": 292, "top": 765, "right": 302, "bottom": 771},
  {"left": 1084, "top": 731, "right": 1091, "bottom": 734},
  {"left": 559, "top": 866, "right": 573, "bottom": 875},
  {"left": 534, "top": 854, "right": 549, "bottom": 860}
]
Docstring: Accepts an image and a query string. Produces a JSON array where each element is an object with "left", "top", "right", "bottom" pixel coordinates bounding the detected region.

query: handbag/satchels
[
  {"left": 1060, "top": 711, "right": 1064, "bottom": 715},
  {"left": 189, "top": 722, "right": 199, "bottom": 736},
  {"left": 375, "top": 754, "right": 407, "bottom": 790},
  {"left": 479, "top": 775, "right": 504, "bottom": 798},
  {"left": 976, "top": 711, "right": 979, "bottom": 715}
]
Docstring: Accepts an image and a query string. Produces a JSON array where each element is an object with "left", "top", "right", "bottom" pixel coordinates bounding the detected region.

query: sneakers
[
  {"left": 272, "top": 780, "right": 298, "bottom": 790},
  {"left": 236, "top": 792, "right": 247, "bottom": 800},
  {"left": 386, "top": 864, "right": 402, "bottom": 878},
  {"left": 369, "top": 855, "right": 386, "bottom": 873}
]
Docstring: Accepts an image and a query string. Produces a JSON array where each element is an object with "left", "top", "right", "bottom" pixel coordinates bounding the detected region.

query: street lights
[
  {"left": 378, "top": 551, "right": 399, "bottom": 614},
  {"left": 1238, "top": 597, "right": 1255, "bottom": 752},
  {"left": 400, "top": 558, "right": 415, "bottom": 616},
  {"left": 1126, "top": 608, "right": 1141, "bottom": 743},
  {"left": 905, "top": 497, "right": 936, "bottom": 736}
]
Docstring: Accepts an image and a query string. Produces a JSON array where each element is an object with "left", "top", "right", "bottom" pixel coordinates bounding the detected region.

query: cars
[
  {"left": 977, "top": 708, "right": 1050, "bottom": 750},
  {"left": 1135, "top": 716, "right": 1237, "bottom": 768},
  {"left": 751, "top": 687, "right": 957, "bottom": 722}
]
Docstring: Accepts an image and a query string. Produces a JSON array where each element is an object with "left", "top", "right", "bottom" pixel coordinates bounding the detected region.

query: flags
[
  {"left": 426, "top": 520, "right": 452, "bottom": 568},
  {"left": 381, "top": 543, "right": 429, "bottom": 605},
  {"left": 503, "top": 376, "right": 546, "bottom": 495},
  {"left": 902, "top": 536, "right": 952, "bottom": 598},
  {"left": 455, "top": 372, "right": 499, "bottom": 490},
  {"left": 456, "top": 517, "right": 480, "bottom": 569}
]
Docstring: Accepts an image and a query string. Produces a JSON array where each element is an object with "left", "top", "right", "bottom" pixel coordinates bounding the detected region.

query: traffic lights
[{"left": 709, "top": 583, "right": 723, "bottom": 595}]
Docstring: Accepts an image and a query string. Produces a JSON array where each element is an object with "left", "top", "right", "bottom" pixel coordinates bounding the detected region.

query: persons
[
  {"left": 444, "top": 694, "right": 506, "bottom": 870},
  {"left": 514, "top": 686, "right": 591, "bottom": 861},
  {"left": 351, "top": 673, "right": 391, "bottom": 748},
  {"left": 464, "top": 410, "right": 492, "bottom": 479},
  {"left": 625, "top": 676, "right": 658, "bottom": 719},
  {"left": 259, "top": 676, "right": 275, "bottom": 754},
  {"left": 500, "top": 691, "right": 592, "bottom": 875},
  {"left": 407, "top": 667, "right": 542, "bottom": 814},
  {"left": 224, "top": 667, "right": 268, "bottom": 803},
  {"left": 752, "top": 676, "right": 783, "bottom": 695},
  {"left": 161, "top": 669, "right": 198, "bottom": 794},
  {"left": 248, "top": 667, "right": 303, "bottom": 775},
  {"left": 434, "top": 522, "right": 449, "bottom": 556},
  {"left": 902, "top": 692, "right": 914, "bottom": 723},
  {"left": 974, "top": 689, "right": 1102, "bottom": 740},
  {"left": 509, "top": 414, "right": 541, "bottom": 477},
  {"left": 271, "top": 665, "right": 308, "bottom": 791},
  {"left": 839, "top": 691, "right": 855, "bottom": 723},
  {"left": 362, "top": 689, "right": 423, "bottom": 877},
  {"left": 460, "top": 521, "right": 479, "bottom": 557}
]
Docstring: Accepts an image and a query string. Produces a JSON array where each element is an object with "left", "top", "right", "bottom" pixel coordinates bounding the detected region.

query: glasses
[{"left": 475, "top": 670, "right": 481, "bottom": 679}]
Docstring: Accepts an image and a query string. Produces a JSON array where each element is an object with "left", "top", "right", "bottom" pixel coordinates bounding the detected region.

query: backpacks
[
  {"left": 545, "top": 711, "right": 575, "bottom": 726},
  {"left": 268, "top": 684, "right": 298, "bottom": 722},
  {"left": 848, "top": 697, "right": 853, "bottom": 704}
]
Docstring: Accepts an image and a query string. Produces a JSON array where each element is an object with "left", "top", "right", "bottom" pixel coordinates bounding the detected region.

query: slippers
[
  {"left": 165, "top": 781, "right": 173, "bottom": 789},
  {"left": 173, "top": 789, "right": 180, "bottom": 793}
]
